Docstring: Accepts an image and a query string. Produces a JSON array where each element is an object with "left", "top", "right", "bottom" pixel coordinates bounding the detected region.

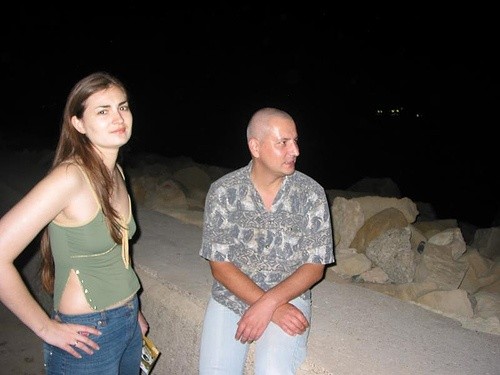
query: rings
[{"left": 74, "top": 341, "right": 78, "bottom": 346}]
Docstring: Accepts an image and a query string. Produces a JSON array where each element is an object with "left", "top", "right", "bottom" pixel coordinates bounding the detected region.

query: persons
[
  {"left": 199, "top": 108, "right": 336, "bottom": 375},
  {"left": 0, "top": 74, "right": 149, "bottom": 375}
]
